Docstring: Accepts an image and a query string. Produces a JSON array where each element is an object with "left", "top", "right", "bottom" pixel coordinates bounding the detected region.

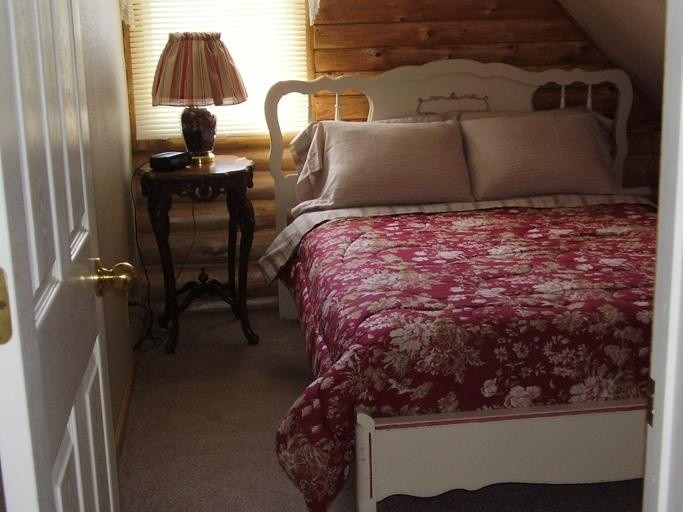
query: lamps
[{"left": 152, "top": 31, "right": 248, "bottom": 165}]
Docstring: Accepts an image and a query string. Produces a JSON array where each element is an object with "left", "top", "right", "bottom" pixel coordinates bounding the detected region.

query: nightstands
[{"left": 139, "top": 154, "right": 259, "bottom": 354}]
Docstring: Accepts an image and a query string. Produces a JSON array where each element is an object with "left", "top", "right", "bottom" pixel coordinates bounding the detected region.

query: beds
[{"left": 257, "top": 58, "right": 659, "bottom": 511}]
